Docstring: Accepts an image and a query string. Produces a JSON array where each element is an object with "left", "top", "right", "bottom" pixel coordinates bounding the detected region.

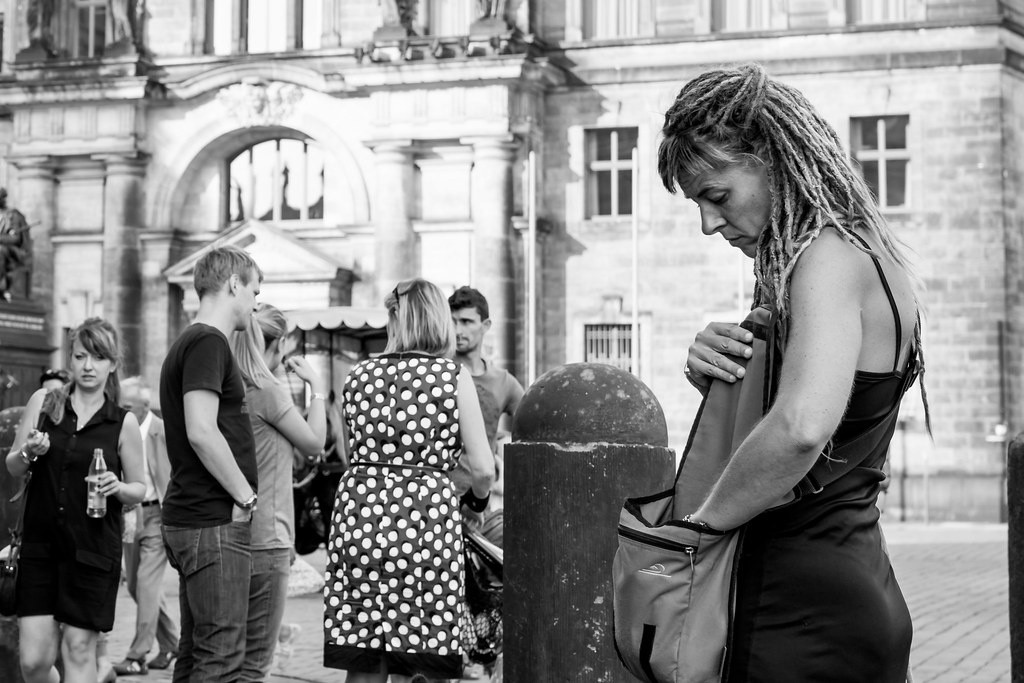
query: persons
[
  {"left": 230, "top": 303, "right": 327, "bottom": 683},
  {"left": 159, "top": 245, "right": 264, "bottom": 683},
  {"left": 41, "top": 369, "right": 73, "bottom": 390},
  {"left": 114, "top": 377, "right": 180, "bottom": 675},
  {"left": 658, "top": 67, "right": 933, "bottom": 683},
  {"left": 323, "top": 275, "right": 496, "bottom": 683},
  {"left": 5, "top": 318, "right": 147, "bottom": 683},
  {"left": 0, "top": 188, "right": 33, "bottom": 298},
  {"left": 448, "top": 287, "right": 525, "bottom": 549}
]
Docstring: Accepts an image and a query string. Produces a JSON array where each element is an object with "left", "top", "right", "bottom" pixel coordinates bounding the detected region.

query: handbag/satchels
[
  {"left": 610, "top": 301, "right": 778, "bottom": 682},
  {"left": 0, "top": 540, "right": 21, "bottom": 618}
]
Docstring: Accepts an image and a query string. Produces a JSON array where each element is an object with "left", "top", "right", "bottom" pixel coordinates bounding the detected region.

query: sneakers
[
  {"left": 146, "top": 650, "right": 180, "bottom": 670},
  {"left": 111, "top": 656, "right": 149, "bottom": 676}
]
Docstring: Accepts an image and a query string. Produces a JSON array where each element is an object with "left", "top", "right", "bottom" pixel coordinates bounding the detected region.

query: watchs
[
  {"left": 236, "top": 493, "right": 258, "bottom": 510},
  {"left": 20, "top": 448, "right": 37, "bottom": 465}
]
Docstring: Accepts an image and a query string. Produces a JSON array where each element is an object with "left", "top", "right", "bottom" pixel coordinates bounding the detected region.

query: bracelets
[
  {"left": 310, "top": 393, "right": 324, "bottom": 401},
  {"left": 682, "top": 514, "right": 708, "bottom": 527},
  {"left": 683, "top": 364, "right": 690, "bottom": 377},
  {"left": 463, "top": 486, "right": 490, "bottom": 513}
]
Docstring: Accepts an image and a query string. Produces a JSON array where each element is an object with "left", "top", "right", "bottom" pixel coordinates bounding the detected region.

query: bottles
[{"left": 86, "top": 448, "right": 107, "bottom": 518}]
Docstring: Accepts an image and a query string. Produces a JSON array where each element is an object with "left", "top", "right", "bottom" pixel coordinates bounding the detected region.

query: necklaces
[{"left": 75, "top": 410, "right": 93, "bottom": 428}]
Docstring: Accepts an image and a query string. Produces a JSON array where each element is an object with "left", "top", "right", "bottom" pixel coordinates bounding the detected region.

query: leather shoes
[{"left": 141, "top": 499, "right": 159, "bottom": 507}]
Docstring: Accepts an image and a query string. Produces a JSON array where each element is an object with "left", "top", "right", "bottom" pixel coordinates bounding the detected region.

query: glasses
[{"left": 45, "top": 369, "right": 70, "bottom": 379}]
